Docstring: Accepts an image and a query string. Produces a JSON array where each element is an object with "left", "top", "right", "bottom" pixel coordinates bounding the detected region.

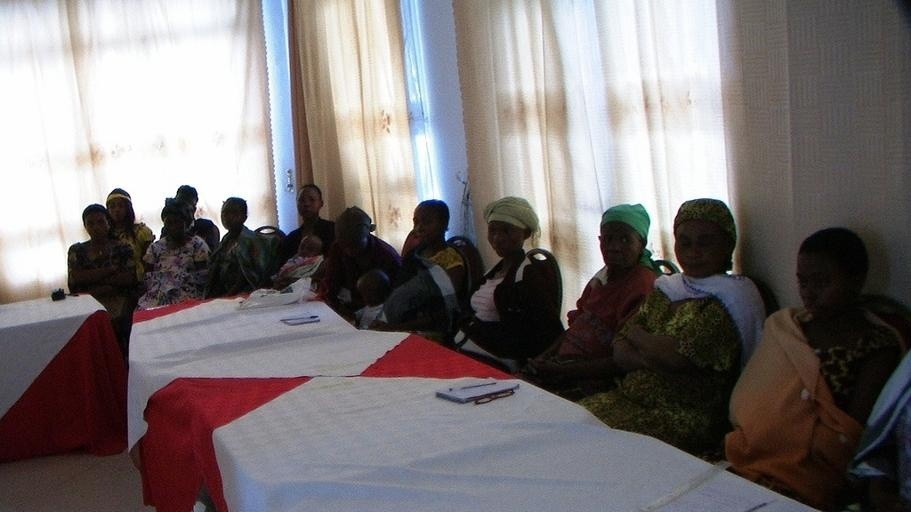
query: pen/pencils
[
  {"left": 747, "top": 499, "right": 775, "bottom": 512},
  {"left": 449, "top": 383, "right": 496, "bottom": 391},
  {"left": 280, "top": 316, "right": 318, "bottom": 321}
]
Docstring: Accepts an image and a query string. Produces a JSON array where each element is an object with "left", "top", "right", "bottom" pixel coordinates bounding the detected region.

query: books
[
  {"left": 283, "top": 312, "right": 320, "bottom": 326},
  {"left": 435, "top": 376, "right": 521, "bottom": 403}
]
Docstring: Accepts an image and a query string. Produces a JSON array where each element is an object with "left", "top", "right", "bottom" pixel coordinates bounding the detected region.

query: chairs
[
  {"left": 523, "top": 247, "right": 566, "bottom": 333},
  {"left": 856, "top": 297, "right": 908, "bottom": 354},
  {"left": 255, "top": 223, "right": 288, "bottom": 249},
  {"left": 195, "top": 221, "right": 220, "bottom": 245},
  {"left": 440, "top": 234, "right": 485, "bottom": 304},
  {"left": 652, "top": 259, "right": 684, "bottom": 274}
]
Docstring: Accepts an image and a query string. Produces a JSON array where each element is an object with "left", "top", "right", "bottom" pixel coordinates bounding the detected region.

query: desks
[
  {"left": 1, "top": 293, "right": 125, "bottom": 461},
  {"left": 125, "top": 288, "right": 830, "bottom": 512}
]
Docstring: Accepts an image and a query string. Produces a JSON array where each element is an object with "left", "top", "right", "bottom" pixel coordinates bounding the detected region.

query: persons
[
  {"left": 574, "top": 199, "right": 766, "bottom": 466},
  {"left": 159, "top": 185, "right": 220, "bottom": 252},
  {"left": 348, "top": 269, "right": 392, "bottom": 332},
  {"left": 451, "top": 198, "right": 566, "bottom": 372},
  {"left": 104, "top": 190, "right": 156, "bottom": 293},
  {"left": 847, "top": 346, "right": 910, "bottom": 511},
  {"left": 368, "top": 200, "right": 470, "bottom": 333},
  {"left": 68, "top": 204, "right": 145, "bottom": 370},
  {"left": 281, "top": 185, "right": 336, "bottom": 253},
  {"left": 273, "top": 235, "right": 322, "bottom": 288},
  {"left": 724, "top": 227, "right": 905, "bottom": 512},
  {"left": 204, "top": 198, "right": 295, "bottom": 295},
  {"left": 317, "top": 206, "right": 401, "bottom": 305},
  {"left": 139, "top": 202, "right": 211, "bottom": 309},
  {"left": 510, "top": 204, "right": 659, "bottom": 401}
]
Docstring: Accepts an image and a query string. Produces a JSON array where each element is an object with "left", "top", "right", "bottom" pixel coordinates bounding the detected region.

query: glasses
[
  {"left": 110, "top": 188, "right": 129, "bottom": 196},
  {"left": 475, "top": 388, "right": 514, "bottom": 405}
]
